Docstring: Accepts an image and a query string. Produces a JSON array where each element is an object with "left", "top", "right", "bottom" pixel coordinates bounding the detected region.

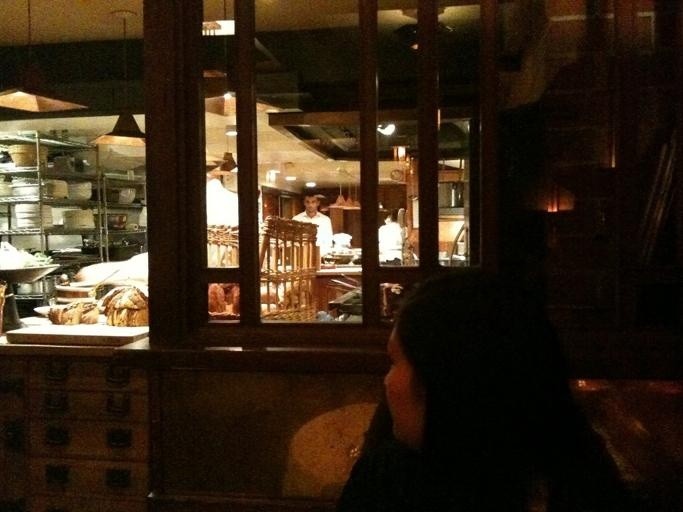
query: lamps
[
  {"left": 87, "top": 8, "right": 146, "bottom": 148},
  {"left": 205, "top": 150, "right": 237, "bottom": 178},
  {"left": 201, "top": 18, "right": 236, "bottom": 36},
  {"left": 0, "top": 0, "right": 88, "bottom": 114},
  {"left": 264, "top": 158, "right": 317, "bottom": 188},
  {"left": 223, "top": 123, "right": 237, "bottom": 137}
]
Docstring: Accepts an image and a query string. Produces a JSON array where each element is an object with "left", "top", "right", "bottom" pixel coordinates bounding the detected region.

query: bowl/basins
[
  {"left": 320, "top": 249, "right": 361, "bottom": 264},
  {"left": 92, "top": 214, "right": 127, "bottom": 230},
  {"left": 32, "top": 304, "right": 62, "bottom": 319},
  {"left": 9, "top": 180, "right": 92, "bottom": 200},
  {"left": 8, "top": 145, "right": 47, "bottom": 166},
  {"left": 108, "top": 187, "right": 135, "bottom": 204},
  {"left": 15, "top": 276, "right": 61, "bottom": 294}
]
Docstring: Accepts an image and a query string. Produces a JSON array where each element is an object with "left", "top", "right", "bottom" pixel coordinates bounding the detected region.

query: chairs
[{"left": 328, "top": 171, "right": 359, "bottom": 213}]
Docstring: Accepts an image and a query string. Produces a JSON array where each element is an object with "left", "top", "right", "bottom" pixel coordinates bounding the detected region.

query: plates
[
  {"left": 14, "top": 204, "right": 95, "bottom": 230},
  {"left": 438, "top": 258, "right": 450, "bottom": 262},
  {"left": 0, "top": 264, "right": 59, "bottom": 284}
]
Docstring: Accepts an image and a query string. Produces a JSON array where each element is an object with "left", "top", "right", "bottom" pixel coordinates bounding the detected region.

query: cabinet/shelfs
[
  {"left": 98, "top": 172, "right": 147, "bottom": 263},
  {"left": 19, "top": 359, "right": 149, "bottom": 510},
  {"left": 0, "top": 133, "right": 102, "bottom": 299}
]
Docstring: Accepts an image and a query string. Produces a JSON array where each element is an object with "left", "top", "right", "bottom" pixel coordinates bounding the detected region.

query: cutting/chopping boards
[{"left": 5, "top": 325, "right": 149, "bottom": 347}]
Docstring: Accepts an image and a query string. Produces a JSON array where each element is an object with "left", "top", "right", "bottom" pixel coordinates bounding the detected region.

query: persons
[
  {"left": 383, "top": 267, "right": 626, "bottom": 512},
  {"left": 291, "top": 188, "right": 333, "bottom": 263}
]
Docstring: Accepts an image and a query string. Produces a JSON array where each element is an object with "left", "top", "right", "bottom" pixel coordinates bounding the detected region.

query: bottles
[
  {"left": 450, "top": 182, "right": 458, "bottom": 209},
  {"left": 47, "top": 129, "right": 70, "bottom": 142}
]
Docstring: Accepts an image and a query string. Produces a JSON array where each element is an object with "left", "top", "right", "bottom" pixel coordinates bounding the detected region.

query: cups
[
  {"left": 457, "top": 243, "right": 466, "bottom": 255},
  {"left": 439, "top": 251, "right": 447, "bottom": 258}
]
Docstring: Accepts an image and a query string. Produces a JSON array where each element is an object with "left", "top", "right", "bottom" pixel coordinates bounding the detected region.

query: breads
[{"left": 46, "top": 269, "right": 280, "bottom": 326}]
